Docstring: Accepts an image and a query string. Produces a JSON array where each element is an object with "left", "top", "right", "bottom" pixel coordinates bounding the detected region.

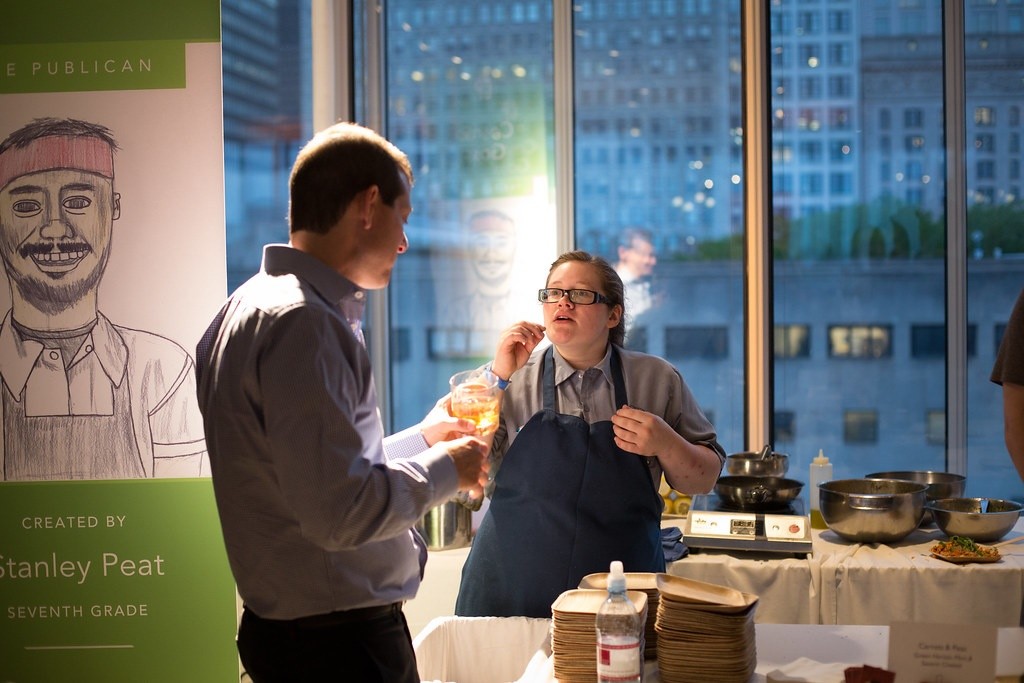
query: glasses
[{"left": 538, "top": 288, "right": 614, "bottom": 305}]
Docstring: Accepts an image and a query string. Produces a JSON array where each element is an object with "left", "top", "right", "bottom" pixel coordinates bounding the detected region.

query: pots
[{"left": 714, "top": 476, "right": 803, "bottom": 508}]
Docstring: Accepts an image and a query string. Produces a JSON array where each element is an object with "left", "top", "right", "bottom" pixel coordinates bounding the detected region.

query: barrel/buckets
[{"left": 422, "top": 501, "right": 472, "bottom": 551}]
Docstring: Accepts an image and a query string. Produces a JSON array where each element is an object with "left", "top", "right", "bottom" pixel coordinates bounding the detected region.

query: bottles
[
  {"left": 595, "top": 560, "right": 644, "bottom": 683},
  {"left": 808, "top": 449, "right": 834, "bottom": 528}
]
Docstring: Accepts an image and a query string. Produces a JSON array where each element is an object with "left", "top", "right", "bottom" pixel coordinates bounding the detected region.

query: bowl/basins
[
  {"left": 924, "top": 498, "right": 1022, "bottom": 544},
  {"left": 817, "top": 480, "right": 930, "bottom": 542},
  {"left": 864, "top": 472, "right": 965, "bottom": 522},
  {"left": 727, "top": 452, "right": 787, "bottom": 477}
]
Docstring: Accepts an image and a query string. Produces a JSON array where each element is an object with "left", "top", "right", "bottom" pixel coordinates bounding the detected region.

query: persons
[
  {"left": 989, "top": 286, "right": 1024, "bottom": 483},
  {"left": 191, "top": 121, "right": 496, "bottom": 683},
  {"left": 455, "top": 252, "right": 725, "bottom": 621},
  {"left": 610, "top": 226, "right": 657, "bottom": 352}
]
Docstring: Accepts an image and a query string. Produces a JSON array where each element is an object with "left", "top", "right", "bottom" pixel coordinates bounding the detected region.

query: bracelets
[{"left": 487, "top": 367, "right": 513, "bottom": 392}]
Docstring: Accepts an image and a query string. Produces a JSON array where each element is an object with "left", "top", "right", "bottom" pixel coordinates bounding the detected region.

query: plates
[
  {"left": 933, "top": 543, "right": 1001, "bottom": 565},
  {"left": 551, "top": 571, "right": 760, "bottom": 683}
]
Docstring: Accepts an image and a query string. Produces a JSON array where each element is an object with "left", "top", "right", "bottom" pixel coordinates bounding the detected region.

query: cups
[{"left": 449, "top": 370, "right": 499, "bottom": 436}]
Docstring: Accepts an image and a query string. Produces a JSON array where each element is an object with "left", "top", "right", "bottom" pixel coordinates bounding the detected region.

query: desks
[
  {"left": 660, "top": 516, "right": 1024, "bottom": 682},
  {"left": 521, "top": 622, "right": 890, "bottom": 683}
]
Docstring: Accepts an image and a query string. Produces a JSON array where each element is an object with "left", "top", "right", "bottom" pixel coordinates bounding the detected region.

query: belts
[{"left": 243, "top": 602, "right": 402, "bottom": 627}]
